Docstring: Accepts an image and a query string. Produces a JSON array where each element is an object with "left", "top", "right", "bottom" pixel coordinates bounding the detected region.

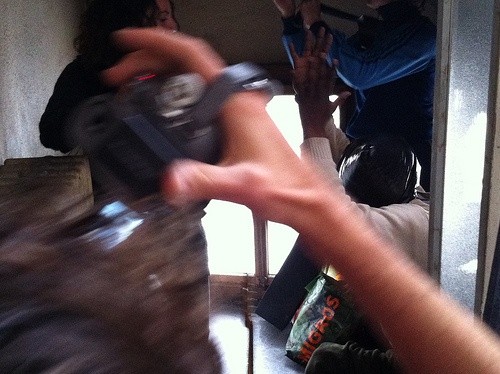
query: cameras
[{"left": 99, "top": 66, "right": 224, "bottom": 206}]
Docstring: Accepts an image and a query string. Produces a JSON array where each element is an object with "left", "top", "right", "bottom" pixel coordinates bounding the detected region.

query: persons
[
  {"left": 104, "top": 27, "right": 500, "bottom": 373},
  {"left": 288, "top": 28, "right": 430, "bottom": 373},
  {"left": 272, "top": 0, "right": 439, "bottom": 193},
  {"left": 39, "top": 0, "right": 211, "bottom": 340}
]
